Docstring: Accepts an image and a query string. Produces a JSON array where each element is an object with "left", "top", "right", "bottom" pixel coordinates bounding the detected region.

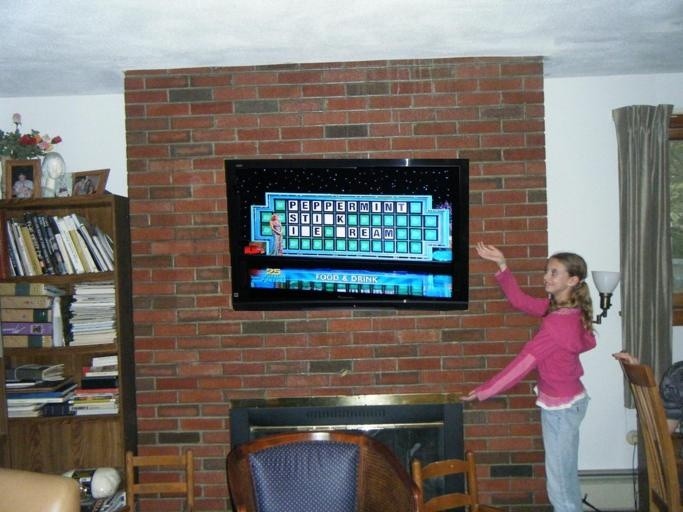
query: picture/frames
[
  {"left": 5, "top": 159, "right": 41, "bottom": 200},
  {"left": 72, "top": 169, "right": 110, "bottom": 200}
]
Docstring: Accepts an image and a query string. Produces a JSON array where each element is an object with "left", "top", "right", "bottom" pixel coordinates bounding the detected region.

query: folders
[{"left": 0, "top": 280, "right": 53, "bottom": 352}]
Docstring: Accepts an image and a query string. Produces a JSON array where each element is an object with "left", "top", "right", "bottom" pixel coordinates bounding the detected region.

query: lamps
[{"left": 590, "top": 271, "right": 620, "bottom": 324}]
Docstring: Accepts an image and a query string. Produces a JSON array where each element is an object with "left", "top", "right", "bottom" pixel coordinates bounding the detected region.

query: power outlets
[{"left": 626, "top": 431, "right": 637, "bottom": 445}]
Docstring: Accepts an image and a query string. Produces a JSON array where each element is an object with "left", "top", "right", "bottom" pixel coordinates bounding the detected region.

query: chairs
[
  {"left": 611, "top": 350, "right": 682, "bottom": 512},
  {"left": 411, "top": 452, "right": 508, "bottom": 511},
  {"left": 225, "top": 430, "right": 420, "bottom": 512},
  {"left": 126, "top": 449, "right": 194, "bottom": 512}
]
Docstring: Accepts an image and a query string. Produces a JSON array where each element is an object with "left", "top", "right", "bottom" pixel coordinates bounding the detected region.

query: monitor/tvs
[{"left": 224, "top": 158, "right": 470, "bottom": 311}]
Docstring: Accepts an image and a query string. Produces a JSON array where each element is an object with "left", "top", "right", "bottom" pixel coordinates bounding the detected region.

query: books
[
  {"left": 63, "top": 467, "right": 127, "bottom": 511},
  {"left": 5, "top": 354, "right": 120, "bottom": 417},
  {"left": 6, "top": 213, "right": 115, "bottom": 277},
  {"left": 0, "top": 280, "right": 118, "bottom": 347}
]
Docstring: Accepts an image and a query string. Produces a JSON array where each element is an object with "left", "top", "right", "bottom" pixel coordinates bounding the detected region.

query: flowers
[{"left": 0, "top": 113, "right": 63, "bottom": 159}]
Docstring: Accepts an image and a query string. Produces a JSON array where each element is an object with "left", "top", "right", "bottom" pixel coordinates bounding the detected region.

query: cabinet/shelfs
[{"left": 1, "top": 194, "right": 130, "bottom": 475}]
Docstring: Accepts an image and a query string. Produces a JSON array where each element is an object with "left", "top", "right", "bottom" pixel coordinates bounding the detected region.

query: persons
[
  {"left": 460, "top": 240, "right": 597, "bottom": 512},
  {"left": 612, "top": 351, "right": 683, "bottom": 440},
  {"left": 13, "top": 174, "right": 34, "bottom": 198}
]
